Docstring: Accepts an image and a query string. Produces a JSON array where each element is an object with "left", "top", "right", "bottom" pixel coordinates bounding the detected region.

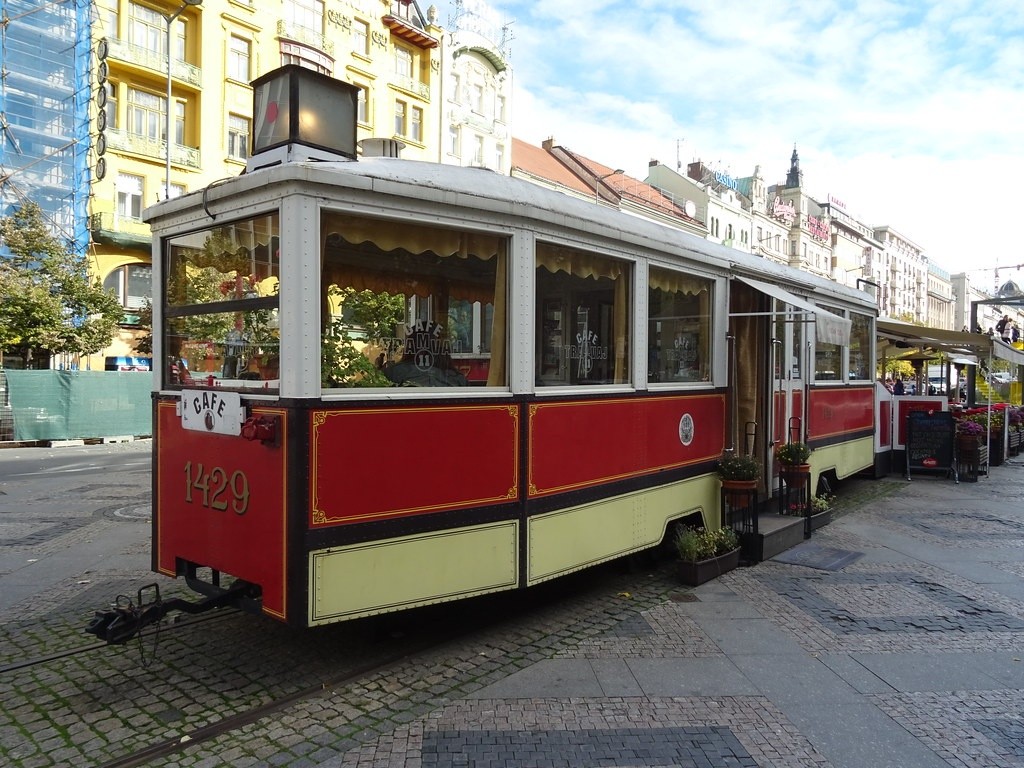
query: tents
[{"left": 877, "top": 316, "right": 1024, "bottom": 477}]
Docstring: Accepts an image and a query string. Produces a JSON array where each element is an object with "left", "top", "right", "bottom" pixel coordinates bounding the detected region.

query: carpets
[{"left": 770, "top": 543, "right": 866, "bottom": 571}]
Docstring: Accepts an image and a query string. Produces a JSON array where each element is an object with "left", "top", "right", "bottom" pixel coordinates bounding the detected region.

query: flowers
[
  {"left": 775, "top": 441, "right": 812, "bottom": 466},
  {"left": 715, "top": 445, "right": 763, "bottom": 480},
  {"left": 958, "top": 420, "right": 984, "bottom": 435},
  {"left": 789, "top": 495, "right": 827, "bottom": 517}
]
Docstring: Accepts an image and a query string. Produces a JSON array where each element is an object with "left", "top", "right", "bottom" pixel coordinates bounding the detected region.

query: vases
[
  {"left": 719, "top": 477, "right": 760, "bottom": 508},
  {"left": 783, "top": 463, "right": 811, "bottom": 488},
  {"left": 959, "top": 434, "right": 980, "bottom": 451},
  {"left": 803, "top": 508, "right": 832, "bottom": 533}
]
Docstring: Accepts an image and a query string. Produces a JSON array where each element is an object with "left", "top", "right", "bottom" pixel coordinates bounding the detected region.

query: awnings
[{"left": 734, "top": 275, "right": 852, "bottom": 347}]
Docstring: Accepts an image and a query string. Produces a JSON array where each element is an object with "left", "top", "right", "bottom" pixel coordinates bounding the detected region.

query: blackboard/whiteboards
[{"left": 908, "top": 411, "right": 953, "bottom": 466}]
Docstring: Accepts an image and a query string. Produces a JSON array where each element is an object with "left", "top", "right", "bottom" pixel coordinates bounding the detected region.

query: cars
[
  {"left": 959, "top": 371, "right": 1012, "bottom": 403},
  {"left": 900, "top": 381, "right": 938, "bottom": 396}
]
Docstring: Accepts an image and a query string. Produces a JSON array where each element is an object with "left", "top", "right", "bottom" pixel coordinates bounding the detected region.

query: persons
[
  {"left": 929, "top": 386, "right": 936, "bottom": 396},
  {"left": 997, "top": 314, "right": 1020, "bottom": 345},
  {"left": 962, "top": 325, "right": 969, "bottom": 332},
  {"left": 977, "top": 322, "right": 994, "bottom": 336},
  {"left": 912, "top": 385, "right": 916, "bottom": 395},
  {"left": 385, "top": 321, "right": 469, "bottom": 387},
  {"left": 886, "top": 377, "right": 904, "bottom": 395}
]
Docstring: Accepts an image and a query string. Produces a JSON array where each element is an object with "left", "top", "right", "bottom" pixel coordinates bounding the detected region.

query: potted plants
[{"left": 671, "top": 520, "right": 742, "bottom": 585}]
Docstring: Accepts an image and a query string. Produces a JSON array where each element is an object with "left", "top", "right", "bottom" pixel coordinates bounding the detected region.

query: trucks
[{"left": 927, "top": 364, "right": 958, "bottom": 402}]
